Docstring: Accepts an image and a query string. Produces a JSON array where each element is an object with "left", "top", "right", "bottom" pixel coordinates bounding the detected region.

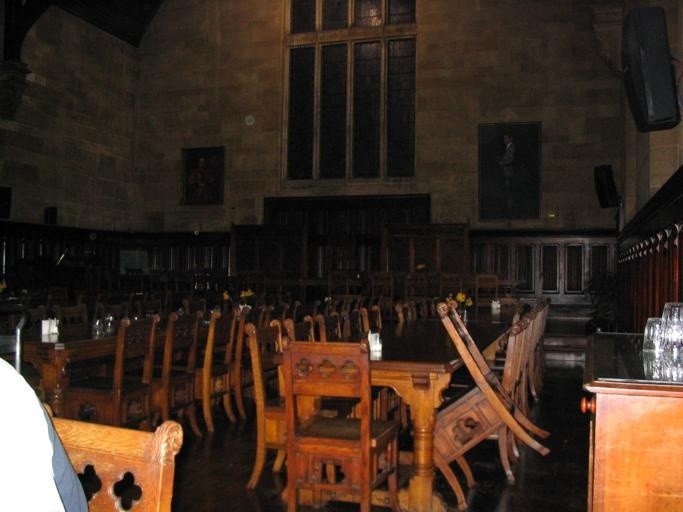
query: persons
[{"left": 499, "top": 127, "right": 520, "bottom": 204}]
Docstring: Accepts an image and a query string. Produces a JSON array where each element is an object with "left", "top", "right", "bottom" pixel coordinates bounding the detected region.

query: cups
[
  {"left": 661, "top": 301, "right": 683, "bottom": 351},
  {"left": 643, "top": 351, "right": 662, "bottom": 380},
  {"left": 642, "top": 317, "right": 662, "bottom": 352},
  {"left": 663, "top": 351, "right": 683, "bottom": 382}
]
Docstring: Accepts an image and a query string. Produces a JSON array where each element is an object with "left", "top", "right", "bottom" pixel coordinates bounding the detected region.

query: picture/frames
[
  {"left": 476, "top": 119, "right": 544, "bottom": 221},
  {"left": 180, "top": 145, "right": 226, "bottom": 205}
]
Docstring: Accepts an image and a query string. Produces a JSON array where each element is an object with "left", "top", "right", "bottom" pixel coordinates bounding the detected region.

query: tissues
[
  {"left": 368, "top": 330, "right": 383, "bottom": 360},
  {"left": 491, "top": 299, "right": 501, "bottom": 314}
]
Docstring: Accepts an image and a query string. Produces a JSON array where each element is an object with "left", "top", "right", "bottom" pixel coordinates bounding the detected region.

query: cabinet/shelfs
[{"left": 582, "top": 331, "right": 683, "bottom": 511}]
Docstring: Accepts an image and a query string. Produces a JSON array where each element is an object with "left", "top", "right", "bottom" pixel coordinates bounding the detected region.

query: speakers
[
  {"left": 593, "top": 165, "right": 619, "bottom": 209},
  {"left": 0, "top": 186, "right": 12, "bottom": 219},
  {"left": 45, "top": 206, "right": 57, "bottom": 224},
  {"left": 621, "top": 6, "right": 681, "bottom": 132}
]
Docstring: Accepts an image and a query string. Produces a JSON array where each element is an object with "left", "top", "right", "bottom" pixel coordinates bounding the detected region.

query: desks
[
  {"left": 0, "top": 321, "right": 165, "bottom": 419},
  {"left": 275, "top": 325, "right": 515, "bottom": 511}
]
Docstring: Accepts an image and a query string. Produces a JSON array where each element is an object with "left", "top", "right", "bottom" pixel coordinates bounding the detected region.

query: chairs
[
  {"left": 284, "top": 339, "right": 400, "bottom": 512},
  {"left": 245, "top": 320, "right": 353, "bottom": 493},
  {"left": 443, "top": 319, "right": 525, "bottom": 486},
  {"left": 431, "top": 295, "right": 552, "bottom": 507},
  {"left": 43, "top": 404, "right": 185, "bottom": 512},
  {"left": 154, "top": 306, "right": 239, "bottom": 430},
  {"left": 64, "top": 312, "right": 161, "bottom": 431},
  {"left": 281, "top": 297, "right": 449, "bottom": 419},
  {"left": 122, "top": 308, "right": 203, "bottom": 439}
]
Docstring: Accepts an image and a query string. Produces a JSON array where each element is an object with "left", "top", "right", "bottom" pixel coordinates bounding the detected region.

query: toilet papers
[{"left": 41, "top": 317, "right": 60, "bottom": 335}]
